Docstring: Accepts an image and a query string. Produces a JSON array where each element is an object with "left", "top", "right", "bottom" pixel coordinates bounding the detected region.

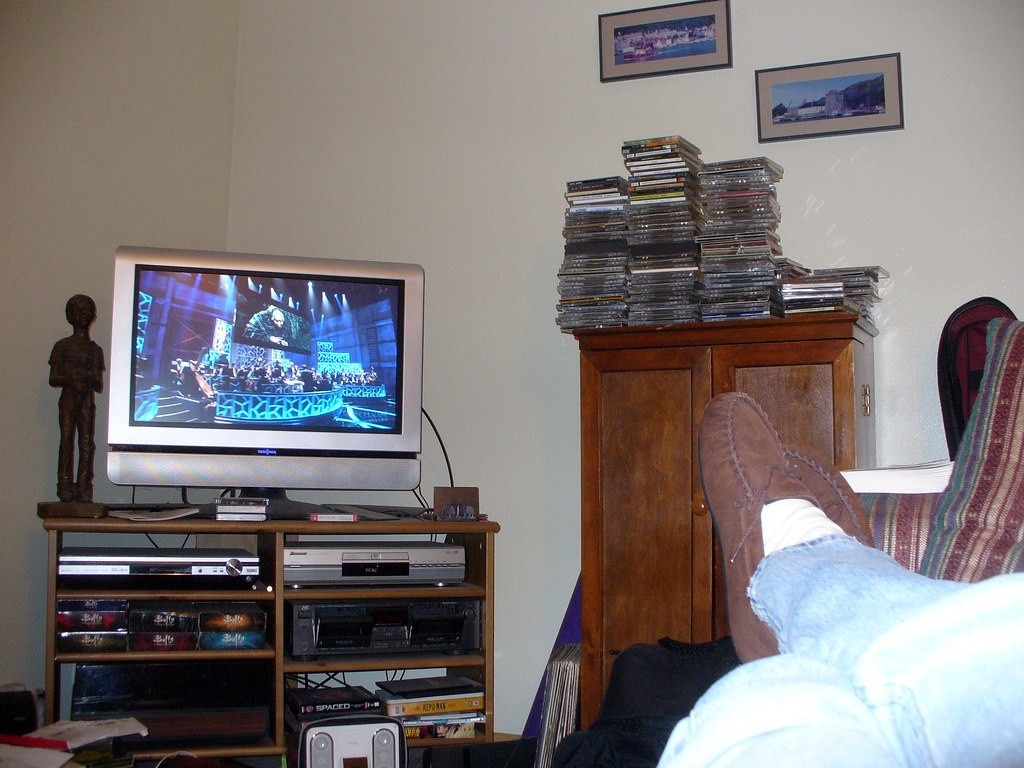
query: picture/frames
[
  {"left": 755, "top": 52, "right": 905, "bottom": 144},
  {"left": 599, "top": 0, "right": 733, "bottom": 83}
]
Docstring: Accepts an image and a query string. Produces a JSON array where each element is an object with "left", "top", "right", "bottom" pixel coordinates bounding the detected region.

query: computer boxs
[{"left": 283, "top": 685, "right": 408, "bottom": 768}]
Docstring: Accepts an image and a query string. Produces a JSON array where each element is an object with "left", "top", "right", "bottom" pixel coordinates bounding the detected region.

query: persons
[
  {"left": 46, "top": 295, "right": 106, "bottom": 503},
  {"left": 659, "top": 391, "right": 1024, "bottom": 768}
]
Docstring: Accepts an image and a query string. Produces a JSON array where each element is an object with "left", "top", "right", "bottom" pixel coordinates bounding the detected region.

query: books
[
  {"left": 400, "top": 712, "right": 486, "bottom": 741},
  {"left": 531, "top": 642, "right": 583, "bottom": 768},
  {"left": 292, "top": 685, "right": 382, "bottom": 715},
  {"left": 308, "top": 513, "right": 360, "bottom": 522},
  {"left": 212, "top": 496, "right": 270, "bottom": 522}
]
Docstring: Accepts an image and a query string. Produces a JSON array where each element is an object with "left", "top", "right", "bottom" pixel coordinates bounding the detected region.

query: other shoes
[
  {"left": 697, "top": 391, "right": 822, "bottom": 666},
  {"left": 778, "top": 441, "right": 876, "bottom": 549}
]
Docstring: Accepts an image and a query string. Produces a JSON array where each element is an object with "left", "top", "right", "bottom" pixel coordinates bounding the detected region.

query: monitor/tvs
[{"left": 108, "top": 245, "right": 425, "bottom": 520}]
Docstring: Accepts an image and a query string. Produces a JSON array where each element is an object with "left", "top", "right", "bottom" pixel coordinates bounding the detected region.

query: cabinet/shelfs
[
  {"left": 576, "top": 315, "right": 879, "bottom": 726},
  {"left": 42, "top": 516, "right": 502, "bottom": 762}
]
